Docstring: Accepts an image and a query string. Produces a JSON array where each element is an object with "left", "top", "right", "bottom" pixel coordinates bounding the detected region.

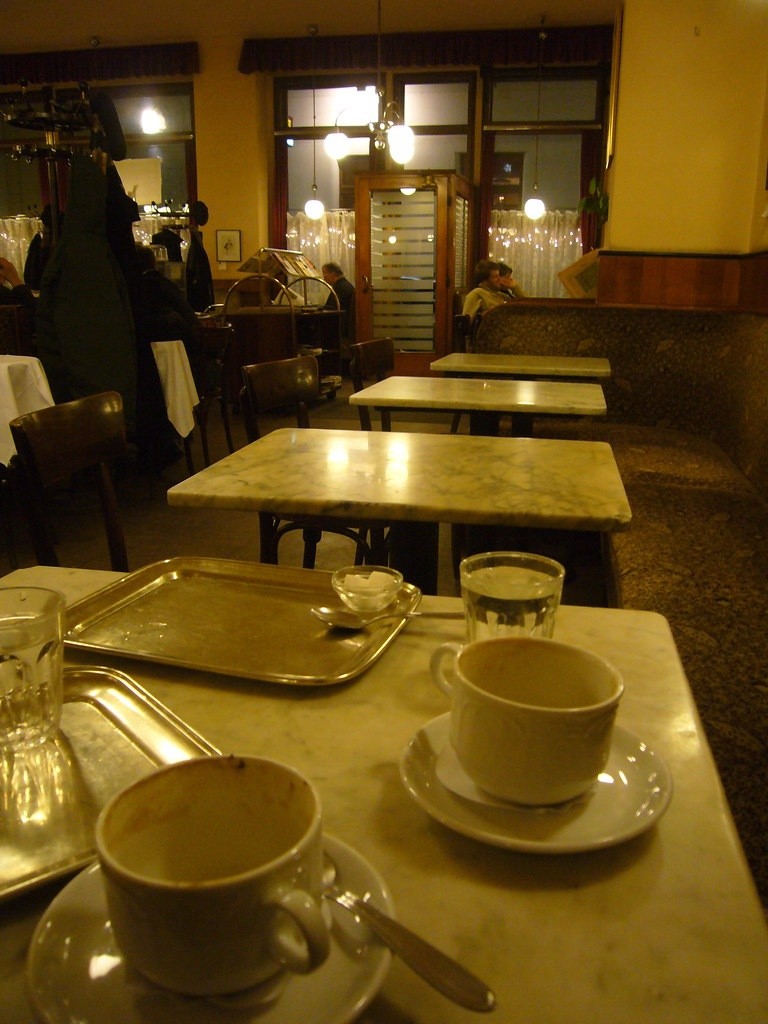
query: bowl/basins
[{"left": 331, "top": 567, "right": 403, "bottom": 611}]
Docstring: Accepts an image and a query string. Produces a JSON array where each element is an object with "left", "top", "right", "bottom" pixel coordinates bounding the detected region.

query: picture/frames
[{"left": 216, "top": 230, "right": 242, "bottom": 262}]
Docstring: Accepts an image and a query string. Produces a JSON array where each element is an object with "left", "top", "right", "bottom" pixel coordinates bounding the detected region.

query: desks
[
  {"left": 0, "top": 566, "right": 768, "bottom": 1024},
  {"left": 144, "top": 338, "right": 200, "bottom": 439},
  {"left": 432, "top": 352, "right": 612, "bottom": 437},
  {"left": 168, "top": 429, "right": 632, "bottom": 599},
  {"left": 350, "top": 366, "right": 607, "bottom": 434},
  {"left": 0, "top": 354, "right": 58, "bottom": 466}
]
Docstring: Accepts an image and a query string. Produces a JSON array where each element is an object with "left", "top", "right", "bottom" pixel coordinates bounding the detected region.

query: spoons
[
  {"left": 323, "top": 853, "right": 496, "bottom": 1013},
  {"left": 309, "top": 607, "right": 464, "bottom": 629}
]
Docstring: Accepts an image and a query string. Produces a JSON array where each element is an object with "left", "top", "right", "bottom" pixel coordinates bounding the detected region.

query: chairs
[
  {"left": 243, "top": 355, "right": 387, "bottom": 581},
  {"left": 194, "top": 322, "right": 234, "bottom": 466},
  {"left": 449, "top": 313, "right": 477, "bottom": 433},
  {"left": 12, "top": 392, "right": 131, "bottom": 567},
  {"left": 352, "top": 338, "right": 437, "bottom": 560}
]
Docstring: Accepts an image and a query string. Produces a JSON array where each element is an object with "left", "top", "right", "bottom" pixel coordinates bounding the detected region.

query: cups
[
  {"left": 460, "top": 551, "right": 565, "bottom": 642},
  {"left": 0, "top": 586, "right": 67, "bottom": 750},
  {"left": 95, "top": 753, "right": 330, "bottom": 995},
  {"left": 431, "top": 637, "right": 625, "bottom": 806}
]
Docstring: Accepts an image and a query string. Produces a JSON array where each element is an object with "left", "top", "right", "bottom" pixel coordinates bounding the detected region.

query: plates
[
  {"left": 26, "top": 831, "right": 391, "bottom": 1023},
  {"left": 401, "top": 710, "right": 671, "bottom": 851}
]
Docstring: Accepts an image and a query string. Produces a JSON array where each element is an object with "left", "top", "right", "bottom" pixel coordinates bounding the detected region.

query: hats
[{"left": 191, "top": 200, "right": 209, "bottom": 226}]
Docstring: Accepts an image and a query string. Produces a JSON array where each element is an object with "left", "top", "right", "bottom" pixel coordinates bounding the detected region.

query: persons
[
  {"left": 120, "top": 247, "right": 203, "bottom": 476},
  {"left": 322, "top": 262, "right": 355, "bottom": 337},
  {"left": 460, "top": 259, "right": 524, "bottom": 347}
]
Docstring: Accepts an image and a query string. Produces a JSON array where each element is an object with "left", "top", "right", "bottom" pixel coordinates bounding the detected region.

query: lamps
[
  {"left": 524, "top": 13, "right": 546, "bottom": 219},
  {"left": 304, "top": 26, "right": 324, "bottom": 219},
  {"left": 323, "top": 0, "right": 417, "bottom": 166}
]
